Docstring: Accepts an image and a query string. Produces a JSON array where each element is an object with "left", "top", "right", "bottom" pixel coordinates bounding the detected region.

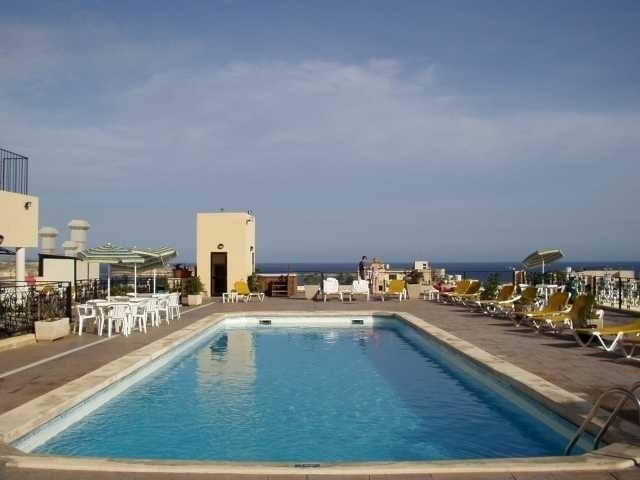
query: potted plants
[
  {"left": 184, "top": 276, "right": 204, "bottom": 306},
  {"left": 303, "top": 273, "right": 321, "bottom": 299}
]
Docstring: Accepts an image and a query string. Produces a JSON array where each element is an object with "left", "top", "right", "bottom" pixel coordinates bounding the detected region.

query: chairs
[
  {"left": 381, "top": 279, "right": 406, "bottom": 301},
  {"left": 234, "top": 281, "right": 265, "bottom": 304},
  {"left": 442, "top": 280, "right": 640, "bottom": 362},
  {"left": 349, "top": 280, "right": 369, "bottom": 302},
  {"left": 73, "top": 292, "right": 182, "bottom": 337},
  {"left": 323, "top": 277, "right": 342, "bottom": 303}
]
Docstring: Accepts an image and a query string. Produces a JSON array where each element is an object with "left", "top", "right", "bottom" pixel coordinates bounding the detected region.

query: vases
[{"left": 34, "top": 317, "right": 69, "bottom": 341}]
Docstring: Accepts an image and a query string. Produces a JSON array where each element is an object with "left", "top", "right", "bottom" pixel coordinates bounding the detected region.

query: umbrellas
[
  {"left": 78, "top": 243, "right": 144, "bottom": 302},
  {"left": 519, "top": 247, "right": 565, "bottom": 308},
  {"left": 131, "top": 245, "right": 176, "bottom": 295},
  {"left": 112, "top": 248, "right": 160, "bottom": 297}
]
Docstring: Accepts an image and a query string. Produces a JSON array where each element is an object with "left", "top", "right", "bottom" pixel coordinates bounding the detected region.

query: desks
[
  {"left": 223, "top": 292, "right": 238, "bottom": 304},
  {"left": 424, "top": 289, "right": 439, "bottom": 301}
]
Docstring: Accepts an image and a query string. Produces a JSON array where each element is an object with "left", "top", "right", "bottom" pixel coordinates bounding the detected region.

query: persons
[
  {"left": 367, "top": 258, "right": 383, "bottom": 294},
  {"left": 359, "top": 256, "right": 367, "bottom": 280}
]
